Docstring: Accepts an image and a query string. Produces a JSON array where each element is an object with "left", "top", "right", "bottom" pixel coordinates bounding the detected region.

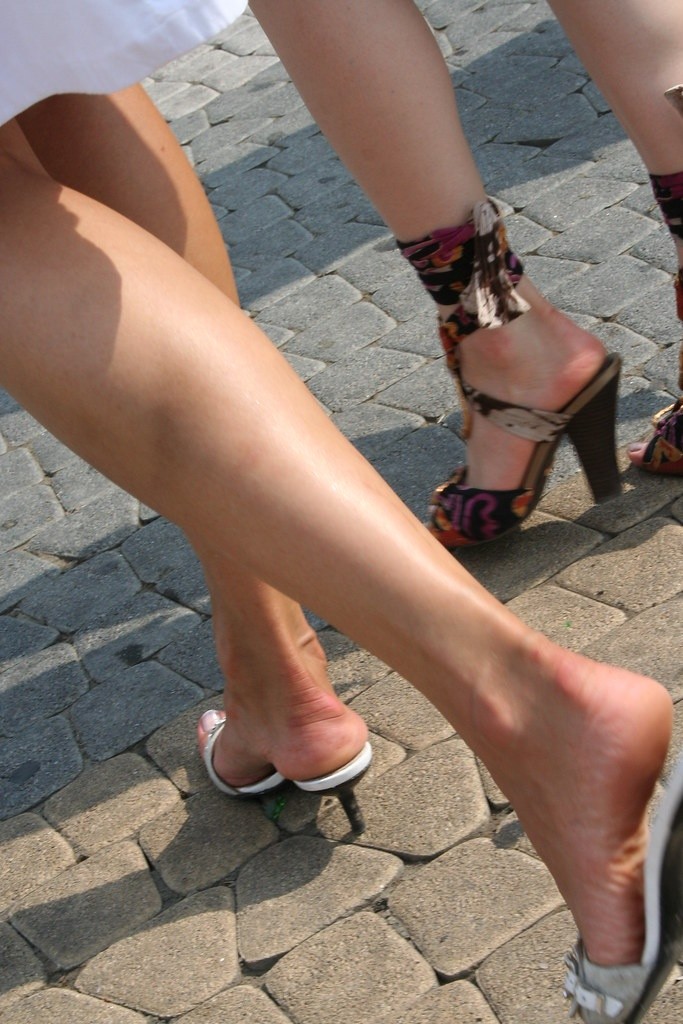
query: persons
[
  {"left": 248, "top": 1, "right": 683, "bottom": 547},
  {"left": 0, "top": 0, "right": 683, "bottom": 1024}
]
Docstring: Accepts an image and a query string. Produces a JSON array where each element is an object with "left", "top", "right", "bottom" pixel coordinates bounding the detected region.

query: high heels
[
  {"left": 203, "top": 715, "right": 374, "bottom": 836},
  {"left": 395, "top": 199, "right": 621, "bottom": 548},
  {"left": 636, "top": 169, "right": 683, "bottom": 475},
  {"left": 562, "top": 764, "right": 683, "bottom": 1024}
]
